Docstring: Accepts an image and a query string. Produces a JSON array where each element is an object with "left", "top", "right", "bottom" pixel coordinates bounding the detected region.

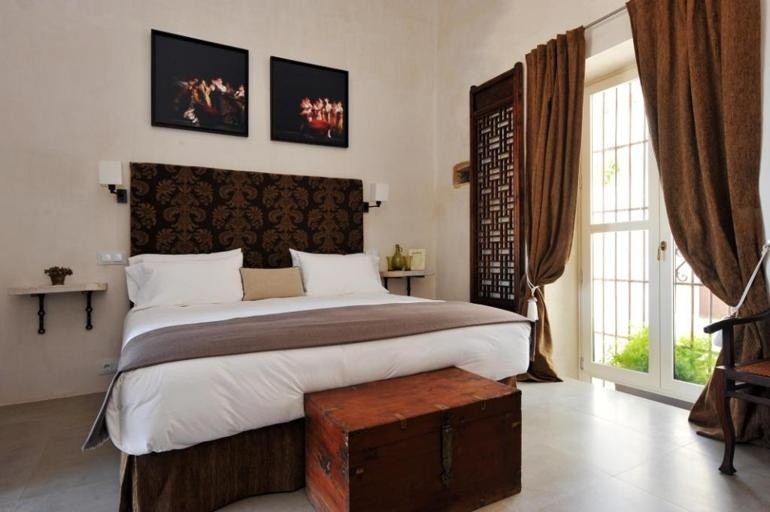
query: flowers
[{"left": 44, "top": 266, "right": 73, "bottom": 276}]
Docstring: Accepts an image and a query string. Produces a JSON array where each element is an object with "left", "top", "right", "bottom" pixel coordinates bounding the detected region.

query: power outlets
[{"left": 95, "top": 356, "right": 112, "bottom": 376}]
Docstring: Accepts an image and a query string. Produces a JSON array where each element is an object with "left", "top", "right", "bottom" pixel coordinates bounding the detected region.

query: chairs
[{"left": 705, "top": 309, "right": 769, "bottom": 474}]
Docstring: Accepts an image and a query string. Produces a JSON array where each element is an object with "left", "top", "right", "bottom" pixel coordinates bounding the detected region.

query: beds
[{"left": 82, "top": 163, "right": 532, "bottom": 512}]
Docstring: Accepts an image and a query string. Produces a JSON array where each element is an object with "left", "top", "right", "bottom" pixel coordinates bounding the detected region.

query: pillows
[
  {"left": 239, "top": 267, "right": 304, "bottom": 300},
  {"left": 288, "top": 245, "right": 390, "bottom": 295},
  {"left": 125, "top": 248, "right": 243, "bottom": 310}
]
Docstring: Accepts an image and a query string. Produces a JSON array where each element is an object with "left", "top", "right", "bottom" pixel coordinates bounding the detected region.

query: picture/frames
[
  {"left": 150, "top": 29, "right": 249, "bottom": 139},
  {"left": 269, "top": 55, "right": 349, "bottom": 150}
]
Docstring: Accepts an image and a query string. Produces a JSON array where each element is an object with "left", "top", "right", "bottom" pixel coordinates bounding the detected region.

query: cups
[
  {"left": 403, "top": 255, "right": 413, "bottom": 272},
  {"left": 387, "top": 256, "right": 396, "bottom": 271}
]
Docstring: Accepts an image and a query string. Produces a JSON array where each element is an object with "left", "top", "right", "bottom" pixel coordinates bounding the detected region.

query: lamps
[
  {"left": 97, "top": 159, "right": 128, "bottom": 204},
  {"left": 364, "top": 181, "right": 389, "bottom": 214}
]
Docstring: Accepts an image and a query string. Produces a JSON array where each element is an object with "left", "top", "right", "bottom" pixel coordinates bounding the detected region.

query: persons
[
  {"left": 297, "top": 94, "right": 346, "bottom": 139},
  {"left": 179, "top": 71, "right": 245, "bottom": 127}
]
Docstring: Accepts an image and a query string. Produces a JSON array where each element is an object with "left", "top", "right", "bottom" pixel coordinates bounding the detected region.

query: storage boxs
[{"left": 304, "top": 365, "right": 522, "bottom": 512}]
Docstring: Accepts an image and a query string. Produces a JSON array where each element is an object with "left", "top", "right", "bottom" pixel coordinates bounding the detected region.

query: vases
[{"left": 52, "top": 276, "right": 65, "bottom": 283}]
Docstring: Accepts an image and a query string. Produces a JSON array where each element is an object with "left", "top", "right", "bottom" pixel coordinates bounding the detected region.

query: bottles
[{"left": 392, "top": 244, "right": 403, "bottom": 270}]
